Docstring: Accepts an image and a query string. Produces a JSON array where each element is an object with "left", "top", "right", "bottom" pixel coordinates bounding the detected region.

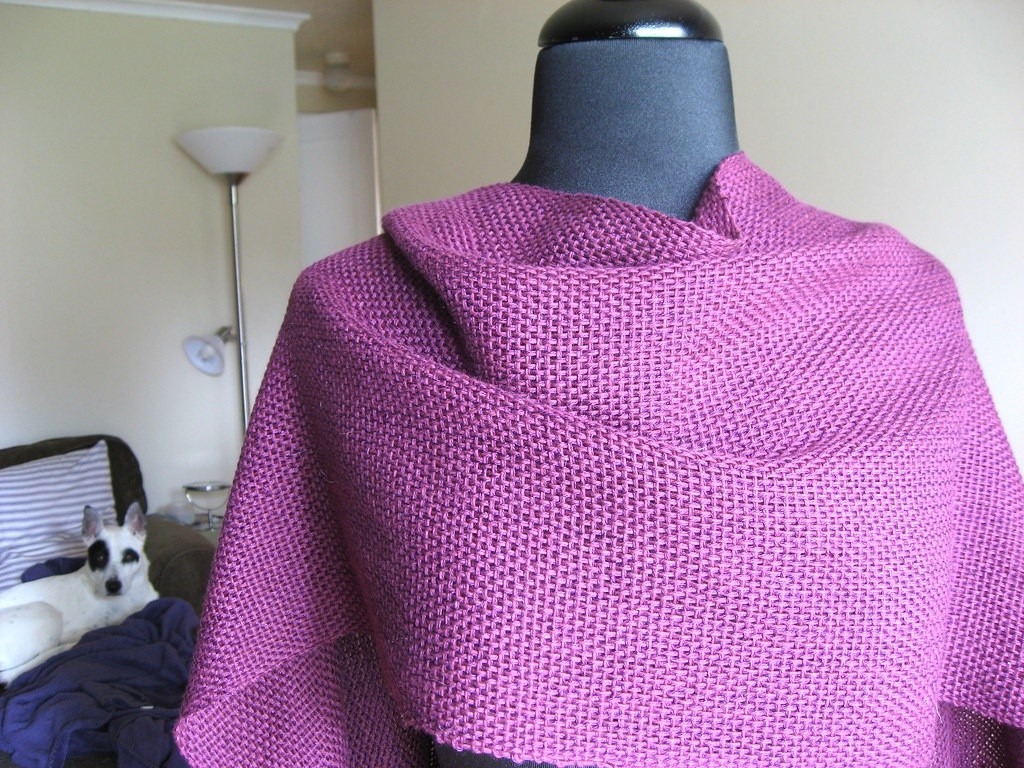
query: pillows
[{"left": 0, "top": 439, "right": 120, "bottom": 597}]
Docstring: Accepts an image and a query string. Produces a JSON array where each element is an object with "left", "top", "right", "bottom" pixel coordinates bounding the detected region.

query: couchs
[{"left": 0, "top": 433, "right": 220, "bottom": 622}]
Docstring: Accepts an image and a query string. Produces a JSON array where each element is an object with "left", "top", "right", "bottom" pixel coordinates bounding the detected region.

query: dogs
[{"left": 0, "top": 502, "right": 161, "bottom": 688}]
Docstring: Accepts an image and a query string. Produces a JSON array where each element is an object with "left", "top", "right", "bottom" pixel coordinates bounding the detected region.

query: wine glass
[{"left": 182, "top": 479, "right": 230, "bottom": 535}]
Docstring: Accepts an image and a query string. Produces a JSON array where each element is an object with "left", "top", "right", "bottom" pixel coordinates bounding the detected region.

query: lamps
[{"left": 172, "top": 123, "right": 288, "bottom": 439}]
